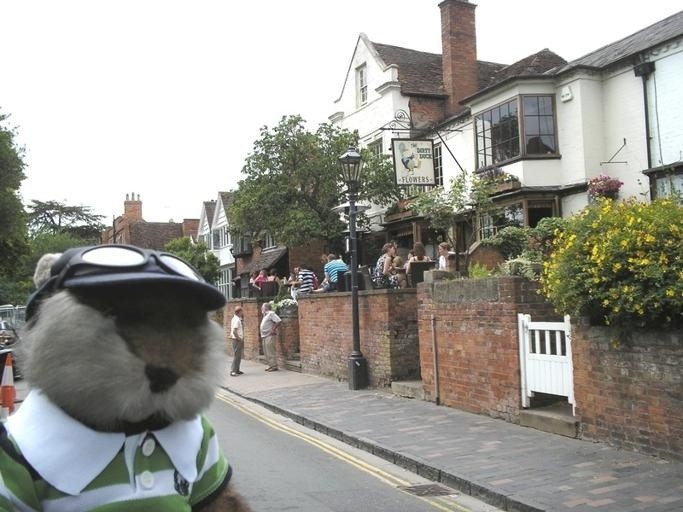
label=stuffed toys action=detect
[1,245,250,511]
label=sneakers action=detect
[230,370,244,376]
[265,366,278,372]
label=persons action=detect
[249,241,450,306]
[230,306,245,376]
[260,303,282,372]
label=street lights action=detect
[336,140,368,390]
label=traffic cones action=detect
[1,351,17,413]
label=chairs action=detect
[407,261,436,286]
[358,265,393,289]
[330,271,345,291]
[247,281,277,297]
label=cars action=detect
[0,306,29,379]
